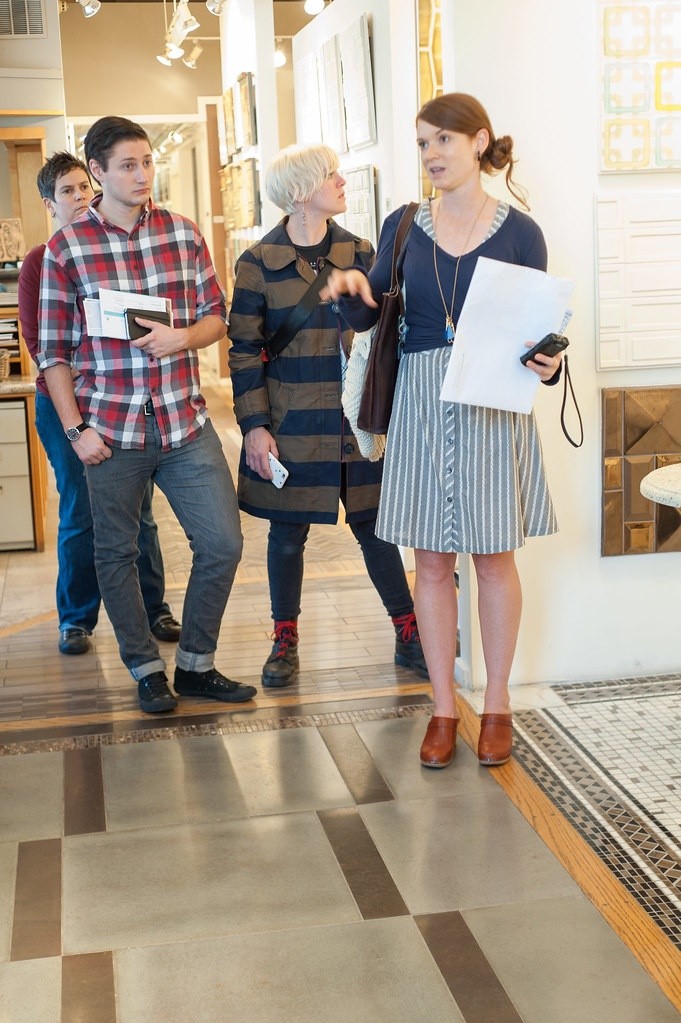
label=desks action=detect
[0,374,48,553]
[640,462,681,509]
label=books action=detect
[124,307,172,340]
[0,318,17,340]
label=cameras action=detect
[519,333,569,367]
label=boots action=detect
[388,605,430,680]
[261,616,300,687]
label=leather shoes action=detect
[419,715,461,767]
[478,713,513,766]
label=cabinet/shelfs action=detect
[0,401,35,553]
[0,306,30,380]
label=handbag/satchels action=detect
[356,201,420,436]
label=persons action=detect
[225,144,438,688]
[18,150,183,656]
[325,93,561,767]
[37,116,257,712]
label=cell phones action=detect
[268,451,289,489]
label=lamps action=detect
[68,0,342,69]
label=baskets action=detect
[0,349,10,382]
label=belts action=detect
[144,401,155,415]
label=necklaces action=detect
[433,192,489,344]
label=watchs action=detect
[67,421,90,442]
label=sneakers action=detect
[58,629,92,654]
[138,671,178,714]
[151,618,182,644]
[172,665,257,700]
